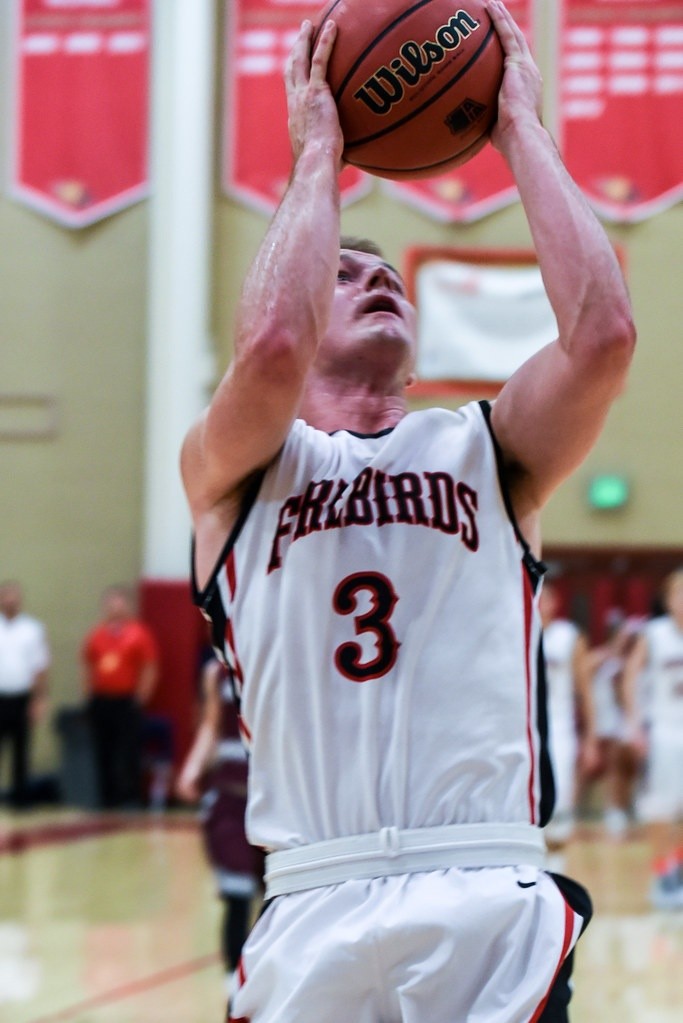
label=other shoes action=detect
[649,845,683,909]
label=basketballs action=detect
[312,0,506,182]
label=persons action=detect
[183,0,637,1023]
[0,581,51,807]
[177,660,268,973]
[538,569,683,907]
[77,586,158,808]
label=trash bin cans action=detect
[49,703,173,812]
